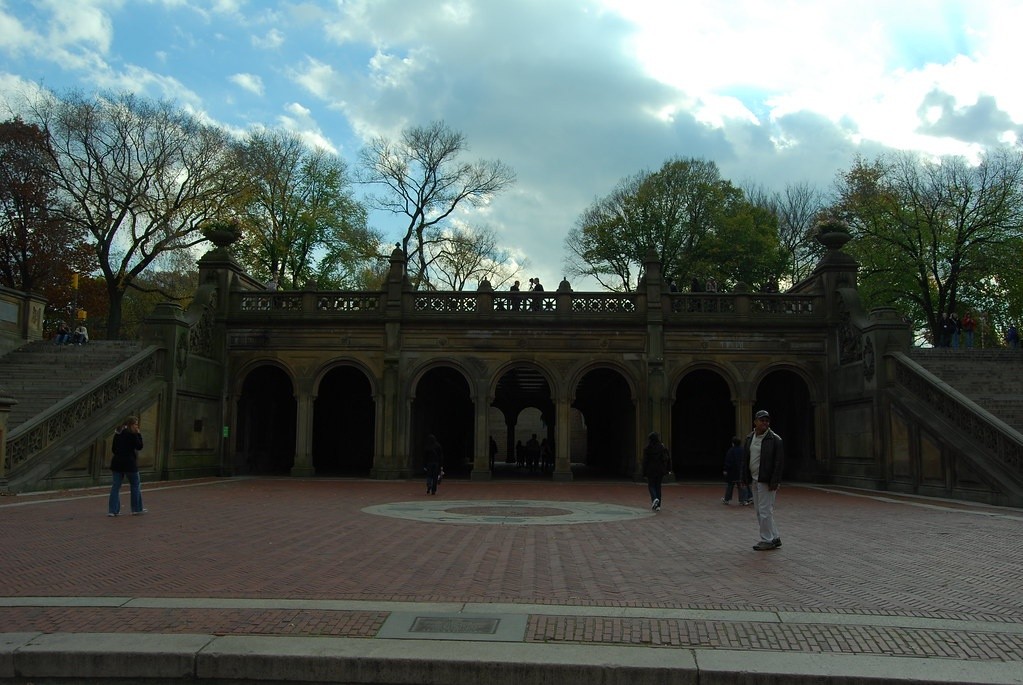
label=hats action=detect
[755,410,769,420]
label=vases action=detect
[821,232,851,249]
[206,230,241,246]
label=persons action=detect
[691,278,702,312]
[509,281,521,311]
[488,435,498,470]
[423,434,442,496]
[720,437,754,505]
[267,286,282,305]
[515,434,551,472]
[938,312,976,350]
[763,276,779,312]
[108,416,144,516]
[666,277,679,292]
[705,276,718,312]
[533,278,544,311]
[641,431,672,512]
[739,410,786,550]
[1004,325,1017,348]
[43,323,89,346]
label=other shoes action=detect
[132,508,148,516]
[427,486,431,494]
[655,506,660,511]
[651,498,659,510]
[738,499,748,506]
[109,510,120,516]
[753,537,782,550]
[721,497,729,505]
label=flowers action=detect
[812,217,857,243]
[198,216,242,232]
